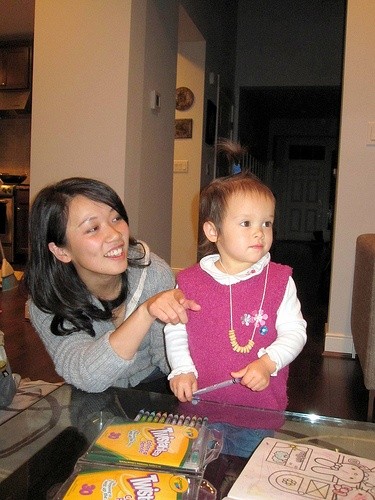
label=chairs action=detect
[350,233,375,421]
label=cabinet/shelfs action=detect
[0,40,33,91]
[15,190,28,264]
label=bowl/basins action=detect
[0,173,28,185]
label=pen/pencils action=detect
[139,408,197,427]
[133,407,145,422]
[194,414,203,431]
[191,416,208,462]
[191,376,241,396]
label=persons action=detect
[163,140,307,458]
[18,178,200,447]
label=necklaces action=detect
[218,251,270,354]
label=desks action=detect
[0,385,375,500]
